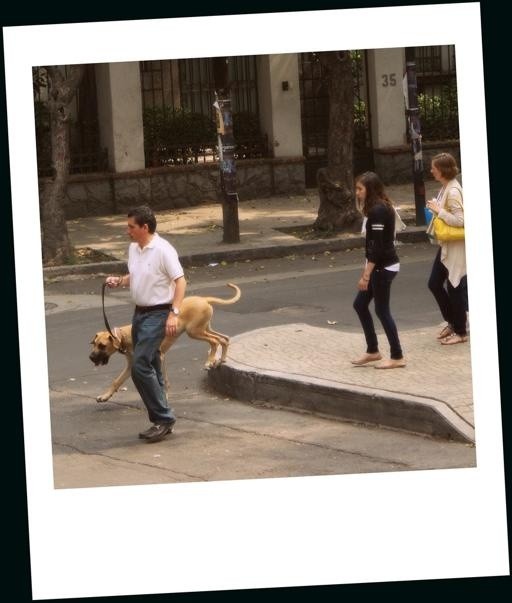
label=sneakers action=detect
[349,351,383,365]
[373,357,407,369]
[144,420,169,442]
[138,423,172,439]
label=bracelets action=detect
[118,274,123,286]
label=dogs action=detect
[88,282,241,403]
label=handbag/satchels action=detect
[431,216,466,242]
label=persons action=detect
[427,153,470,346]
[352,171,407,370]
[106,203,188,443]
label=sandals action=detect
[440,332,468,346]
[436,324,455,340]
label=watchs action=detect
[169,307,181,314]
